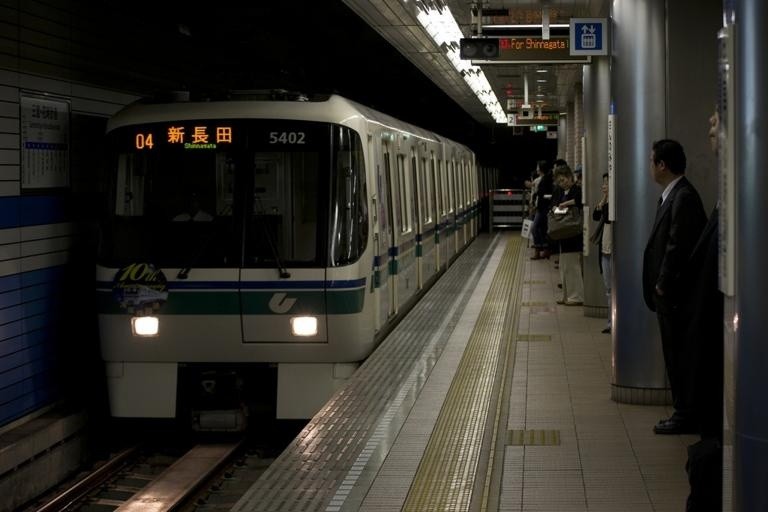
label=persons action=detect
[588,173,613,334]
[687,101,722,448]
[642,138,709,436]
[521,157,582,260]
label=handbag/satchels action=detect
[520,218,535,240]
[545,205,583,241]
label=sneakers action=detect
[652,411,698,437]
[529,249,583,307]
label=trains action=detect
[81,79,508,451]
